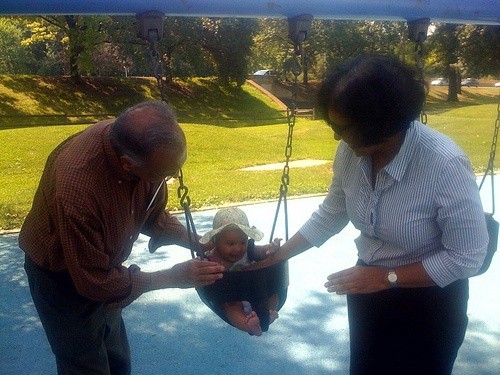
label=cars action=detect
[461,78,480,87]
[431,77,449,86]
[253,69,284,81]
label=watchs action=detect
[246,54,488,374]
[385,267,399,291]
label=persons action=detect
[19,101,223,374]
[196,206,289,337]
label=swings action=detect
[406,17,500,277]
[133,10,316,333]
